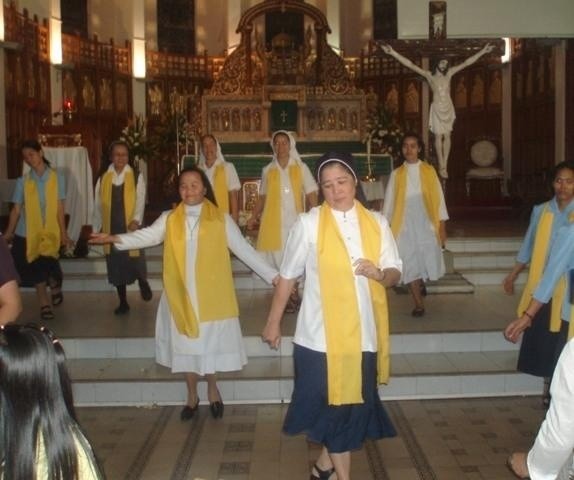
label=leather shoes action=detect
[420,279,427,296]
[412,307,425,317]
[114,303,129,314]
[139,281,152,301]
[181,397,200,420]
[210,389,224,418]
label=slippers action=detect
[507,451,530,479]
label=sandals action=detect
[40,305,54,320]
[310,463,335,480]
[50,283,62,305]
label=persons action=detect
[247,130,319,314]
[197,134,242,221]
[1,321,106,480]
[380,43,497,178]
[93,141,152,317]
[503,160,574,408]
[382,133,450,315]
[505,337,574,480]
[504,210,574,343]
[261,150,402,479]
[87,167,297,421]
[3,140,73,319]
[0,233,25,332]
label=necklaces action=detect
[184,217,201,244]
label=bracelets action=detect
[378,272,387,285]
[523,310,537,321]
